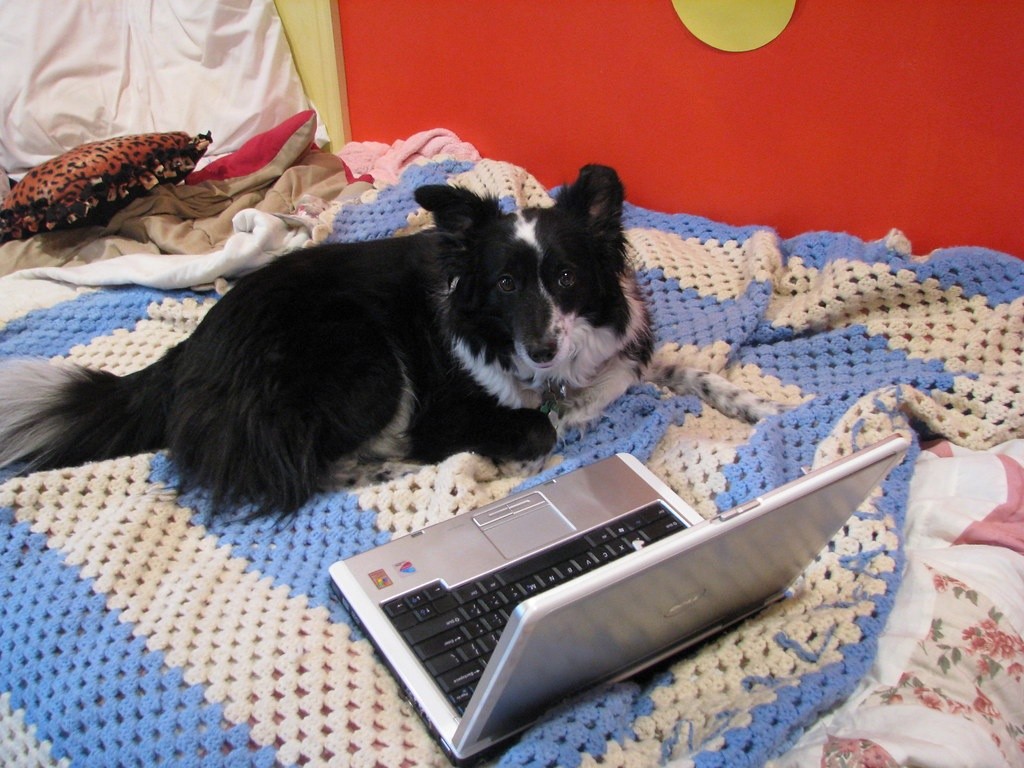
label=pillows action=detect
[0,129,214,244]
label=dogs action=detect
[0,163,659,540]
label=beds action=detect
[0,111,1024,768]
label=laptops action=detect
[327,430,909,768]
